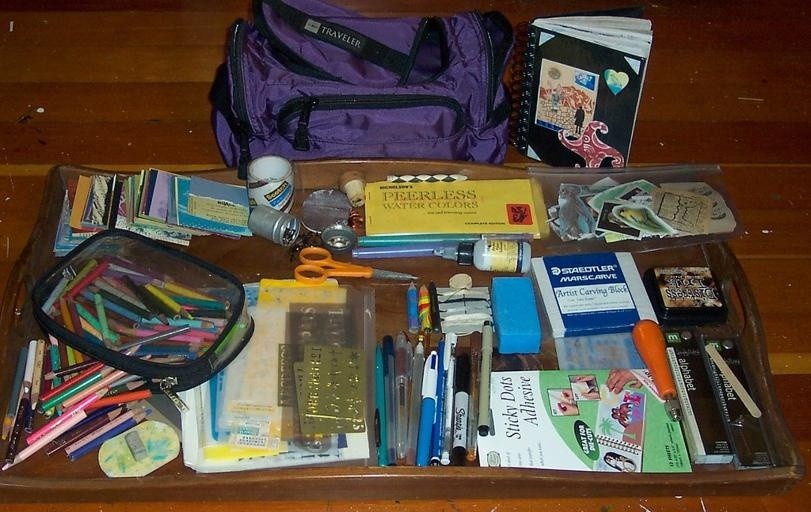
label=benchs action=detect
[2,0,811,389]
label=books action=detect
[515,15,655,168]
[54,168,253,258]
[477,368,693,475]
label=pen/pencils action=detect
[405,278,442,334]
[374,320,495,467]
[42,255,233,365]
[2,338,152,473]
[352,234,535,259]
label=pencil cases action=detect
[31,228,255,393]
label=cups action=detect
[246,153,297,216]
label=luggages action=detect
[205,1,517,170]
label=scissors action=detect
[294,246,420,284]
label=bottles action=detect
[432,237,533,275]
[248,203,301,247]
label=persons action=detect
[556,390,578,414]
[605,368,641,393]
[576,376,600,399]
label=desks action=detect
[1,156,804,504]
